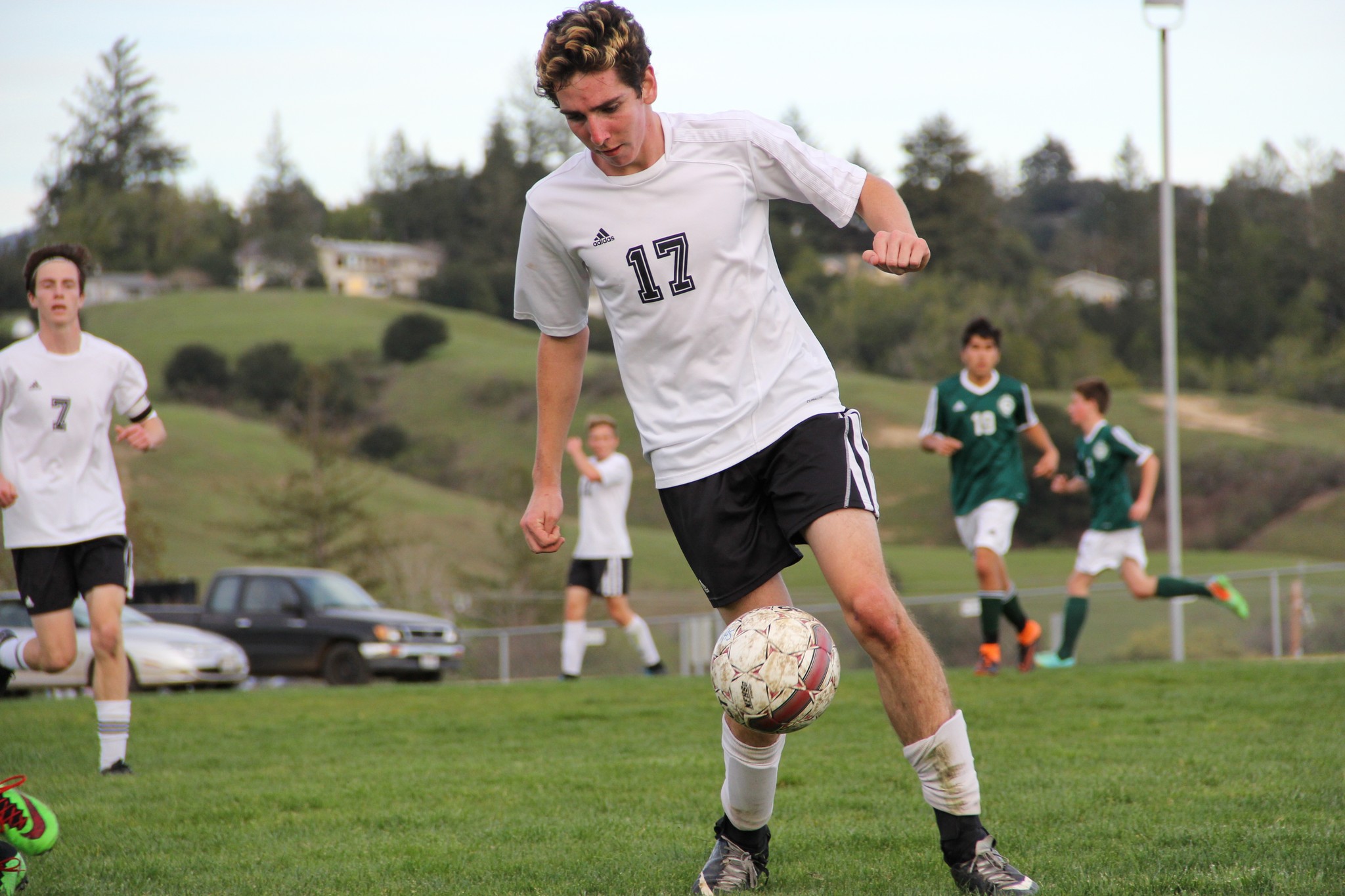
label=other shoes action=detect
[0,629,18,695]
[103,760,132,775]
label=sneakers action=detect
[1016,619,1041,672]
[691,818,771,895]
[0,840,29,896]
[0,776,60,856]
[1207,575,1250,622]
[1034,652,1074,669]
[973,641,1004,675]
[951,833,1039,894]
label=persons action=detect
[1,240,165,896]
[1029,379,1246,668]
[561,415,669,679]
[919,316,1060,682]
[513,1,1035,895]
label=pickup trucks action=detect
[127,565,465,688]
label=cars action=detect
[0,587,249,697]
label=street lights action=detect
[1138,2,1192,667]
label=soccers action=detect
[706,606,840,736]
